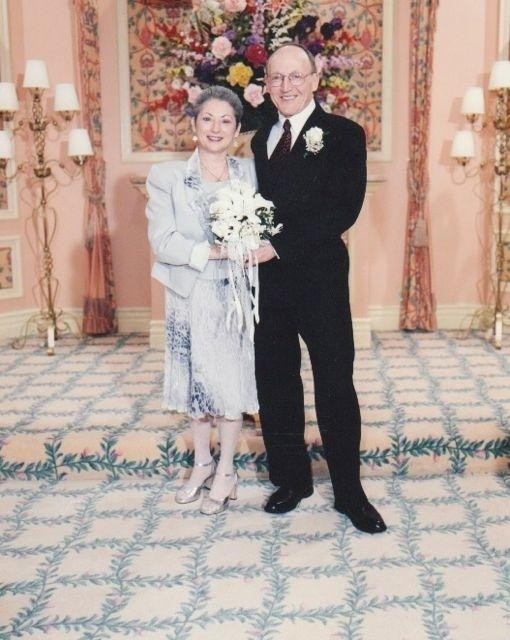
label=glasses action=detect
[270,72,312,86]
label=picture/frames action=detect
[115,0,396,162]
[2,234,25,298]
[0,155,17,218]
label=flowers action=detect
[205,180,284,264]
[148,1,364,123]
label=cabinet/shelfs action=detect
[131,176,390,350]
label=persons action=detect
[145,84,259,514]
[247,40,386,533]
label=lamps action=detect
[449,57,510,348]
[2,55,95,355]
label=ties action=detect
[269,120,290,162]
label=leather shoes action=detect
[265,486,313,513]
[334,499,386,532]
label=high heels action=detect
[201,467,237,514]
[175,457,216,503]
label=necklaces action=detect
[200,161,230,183]
